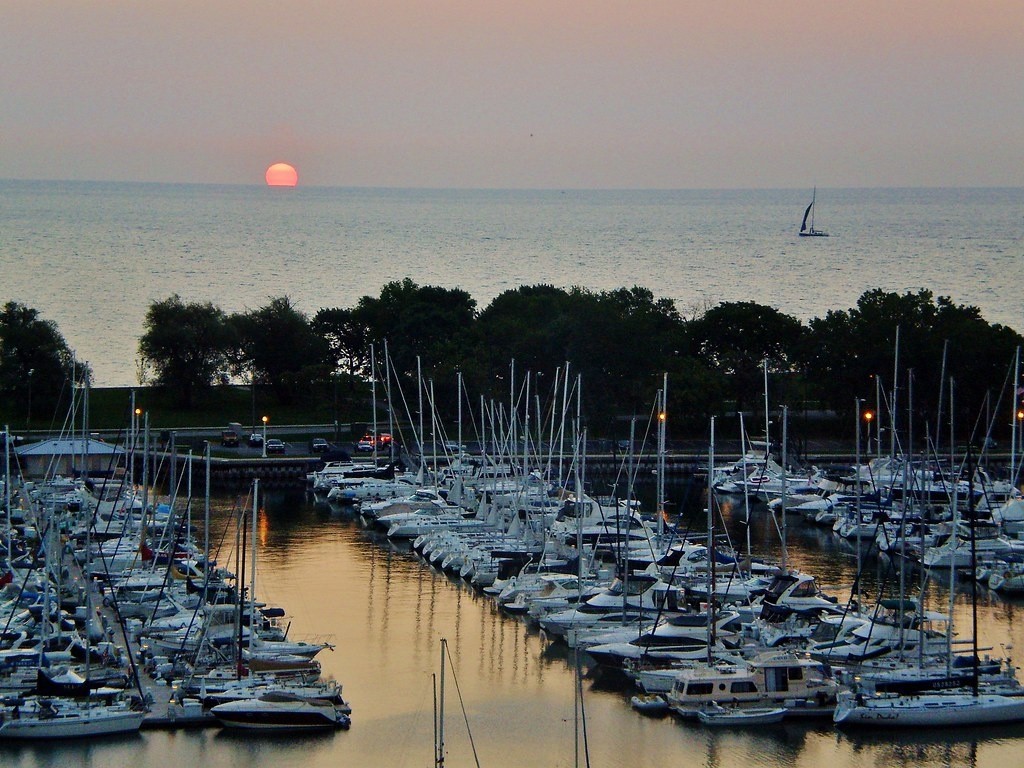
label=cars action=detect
[266,439,286,454]
[441,441,459,452]
[248,433,264,447]
[313,439,328,452]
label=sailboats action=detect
[0,349,338,742]
[298,340,1023,728]
[797,187,829,237]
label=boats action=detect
[211,691,337,734]
[631,693,668,714]
[710,323,1023,597]
[695,689,788,728]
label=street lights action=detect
[1018,413,1024,452]
[866,413,872,454]
[261,416,268,458]
[135,409,142,449]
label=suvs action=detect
[221,429,239,447]
